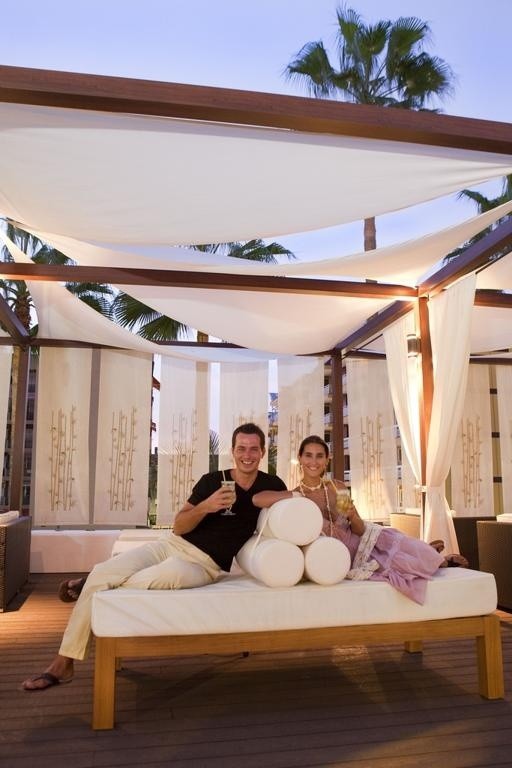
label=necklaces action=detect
[299,478,333,537]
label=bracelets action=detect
[293,489,300,500]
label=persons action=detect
[252,435,469,608]
[18,423,288,691]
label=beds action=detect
[91,527,505,732]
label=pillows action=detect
[304,535,352,586]
[0,510,20,524]
[496,512,512,522]
[257,497,324,546]
[236,538,305,587]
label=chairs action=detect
[389,510,497,568]
[476,519,512,609]
[0,516,30,613]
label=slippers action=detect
[442,551,469,568]
[429,539,446,552]
[22,667,73,692]
[58,576,85,603]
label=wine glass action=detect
[220,480,238,517]
[333,489,352,526]
